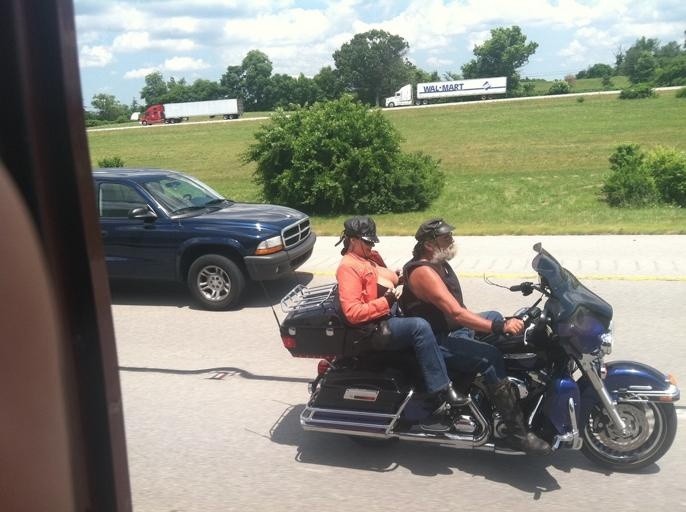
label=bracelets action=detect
[491,318,506,335]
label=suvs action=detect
[86,166,319,313]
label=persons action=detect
[332,215,474,410]
[396,216,552,459]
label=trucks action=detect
[140,97,243,126]
[384,76,508,109]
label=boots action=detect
[488,380,552,456]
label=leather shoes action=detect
[439,387,469,406]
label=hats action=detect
[343,217,380,244]
[415,219,455,240]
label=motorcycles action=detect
[273,237,682,476]
